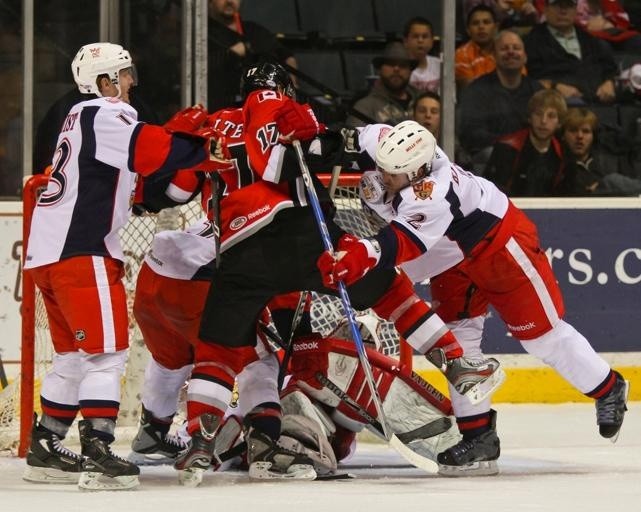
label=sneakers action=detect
[438,409,500,465]
[426,348,499,394]
[596,371,626,438]
[27,403,314,475]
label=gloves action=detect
[275,103,324,142]
[317,233,378,289]
[195,128,233,169]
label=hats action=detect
[373,32,418,70]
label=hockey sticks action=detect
[293,139,439,475]
[259,324,452,442]
[212,165,340,463]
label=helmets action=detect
[376,120,437,181]
[71,43,132,94]
[241,63,295,98]
